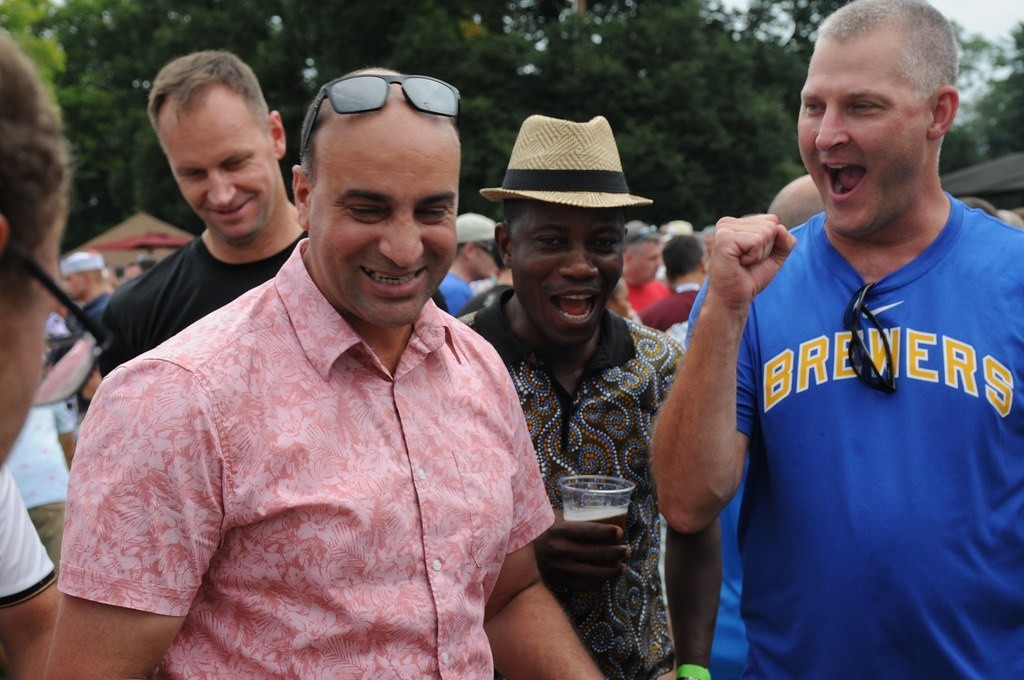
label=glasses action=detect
[10,247,111,407]
[302,73,461,149]
[843,283,898,394]
[630,225,659,242]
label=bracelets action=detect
[676,663,711,680]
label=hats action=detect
[59,251,103,275]
[478,114,654,209]
[661,220,694,243]
[455,212,496,243]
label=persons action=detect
[657,0,1024,680]
[44,202,1024,334]
[53,66,608,680]
[685,174,824,679]
[0,474,61,679]
[451,114,723,680]
[5,377,82,574]
[0,29,113,471]
[101,49,449,379]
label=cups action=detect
[557,475,636,542]
